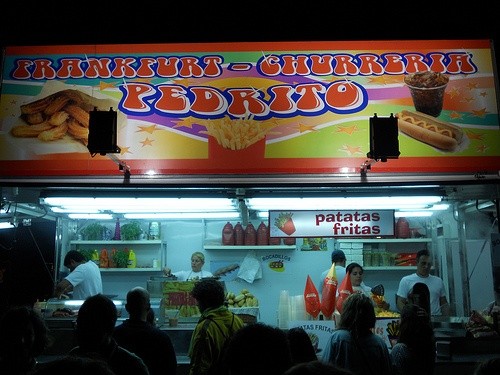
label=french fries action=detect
[11,91,91,141]
[194,113,281,151]
[273,211,294,228]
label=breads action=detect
[227,288,258,307]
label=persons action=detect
[0,307,112,375]
[321,294,393,373]
[396,248,450,317]
[336,263,386,314]
[202,323,354,375]
[318,249,347,315]
[390,307,438,370]
[113,290,177,375]
[189,280,248,375]
[56,250,103,299]
[65,295,149,375]
[163,252,214,281]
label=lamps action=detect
[0,218,18,229]
[87,108,131,176]
[360,111,402,173]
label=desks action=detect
[287,318,401,362]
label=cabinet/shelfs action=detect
[68,238,167,274]
[333,236,435,271]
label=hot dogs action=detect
[395,110,464,149]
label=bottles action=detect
[243,222,257,245]
[396,218,409,238]
[127,249,136,268]
[269,226,281,245]
[255,221,268,245]
[108,249,117,268]
[99,248,109,268]
[90,249,101,267]
[284,237,296,245]
[222,221,235,245]
[233,222,244,245]
[124,248,128,253]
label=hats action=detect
[332,250,347,261]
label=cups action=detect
[153,259,160,268]
[409,229,417,238]
[278,289,310,330]
[362,252,392,266]
[404,76,449,117]
